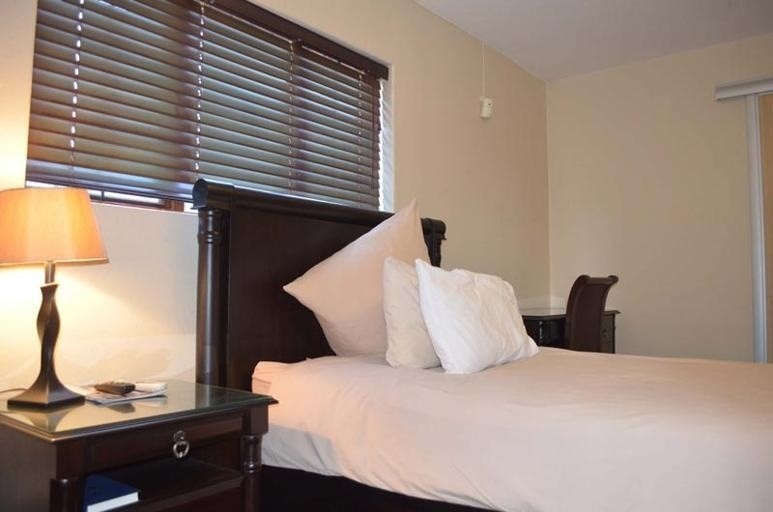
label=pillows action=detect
[284,197,431,363]
[416,257,541,374]
[381,256,443,369]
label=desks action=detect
[521,309,622,353]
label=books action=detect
[81,475,141,511]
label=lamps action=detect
[0,185,110,411]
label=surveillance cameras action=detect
[478,94,495,123]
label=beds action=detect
[193,176,773,511]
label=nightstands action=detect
[2,381,277,511]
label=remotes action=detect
[93,381,135,395]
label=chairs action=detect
[556,275,619,354]
